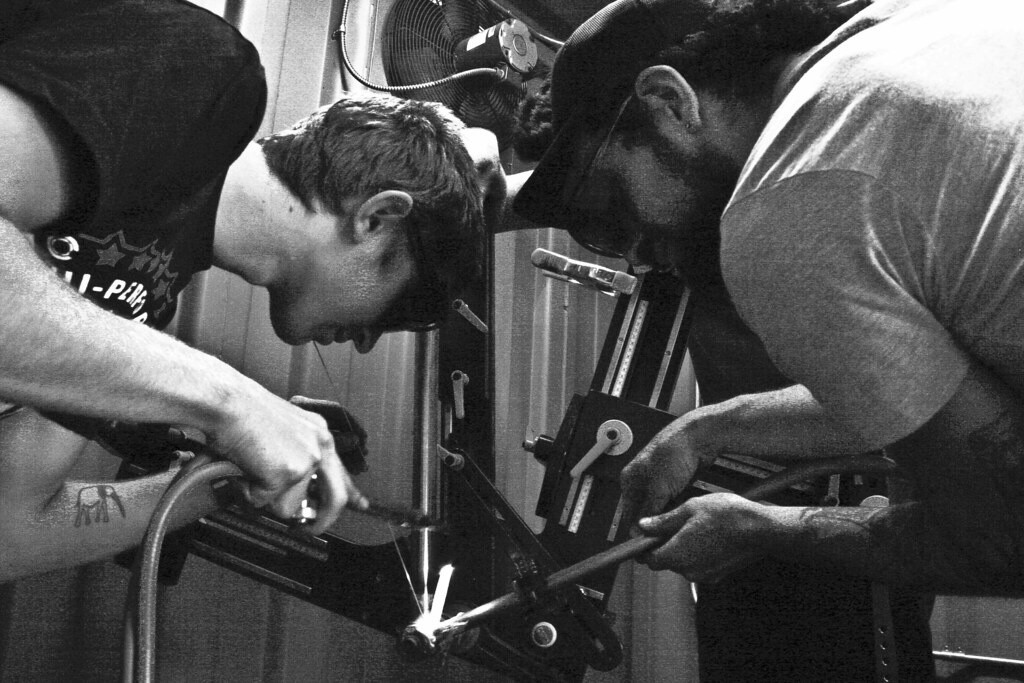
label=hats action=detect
[514,0,712,227]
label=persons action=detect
[0,0,486,583]
[513,2,1024,590]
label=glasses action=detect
[400,210,448,332]
[565,88,638,259]
